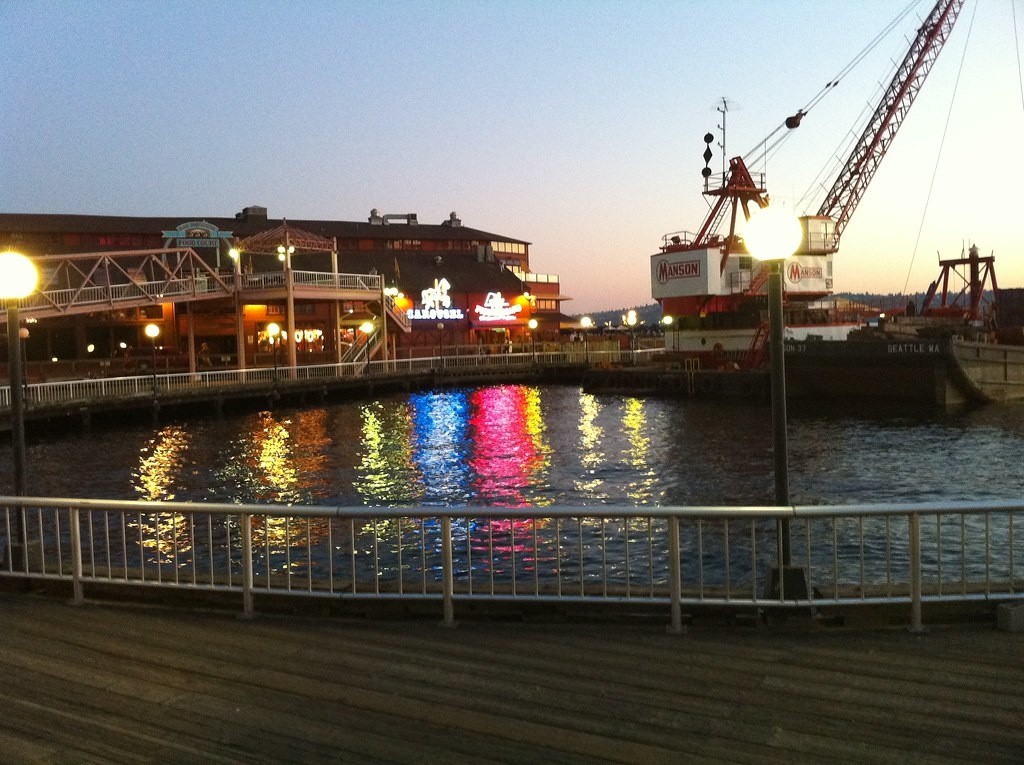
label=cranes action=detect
[649,1,965,325]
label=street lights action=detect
[581,316,591,367]
[267,322,281,390]
[0,248,40,567]
[626,315,638,367]
[527,318,538,369]
[141,322,162,408]
[362,321,374,380]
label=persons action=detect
[574,331,580,342]
[124,345,137,371]
[905,301,915,318]
[501,337,509,365]
[198,342,213,368]
[478,343,492,365]
